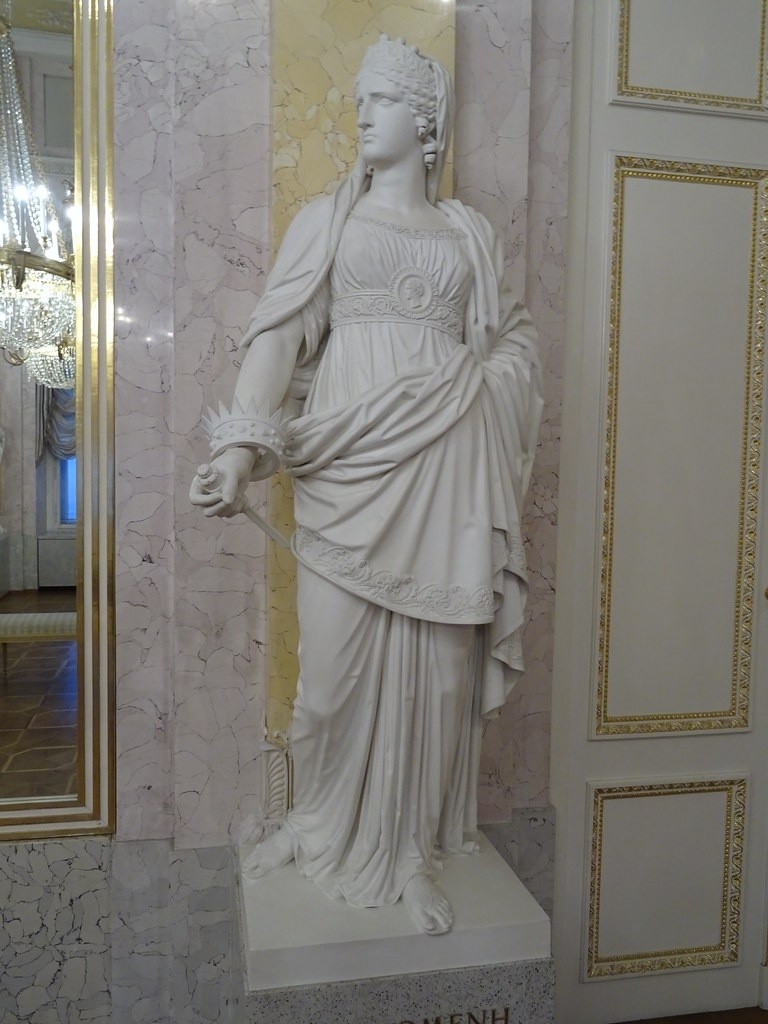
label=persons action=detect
[188,34,544,938]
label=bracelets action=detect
[200,395,298,482]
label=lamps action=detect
[0,12,76,389]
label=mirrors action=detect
[0,0,119,842]
